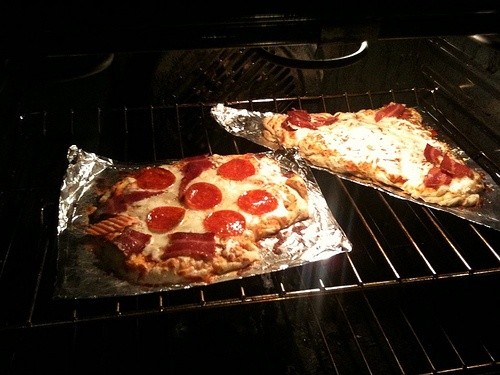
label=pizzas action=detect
[87,152,309,285]
[260,101,485,207]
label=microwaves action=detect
[0,1,500,375]
